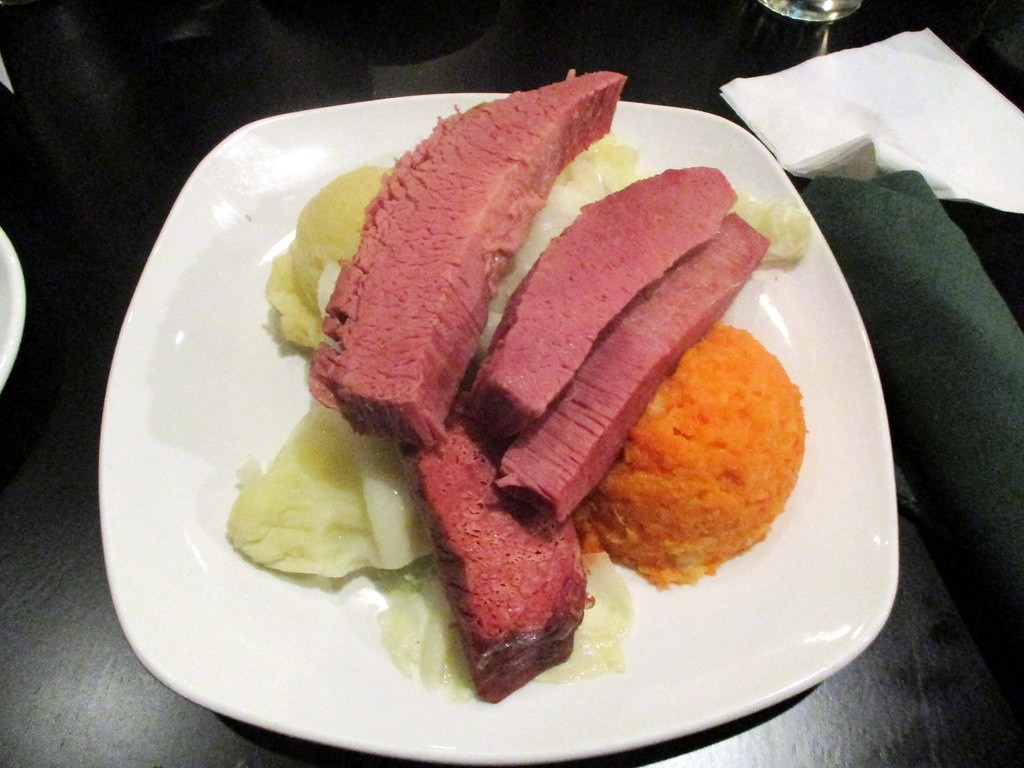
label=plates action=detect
[99,93,898,767]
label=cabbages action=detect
[221,131,810,700]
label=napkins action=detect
[719,28,1024,215]
